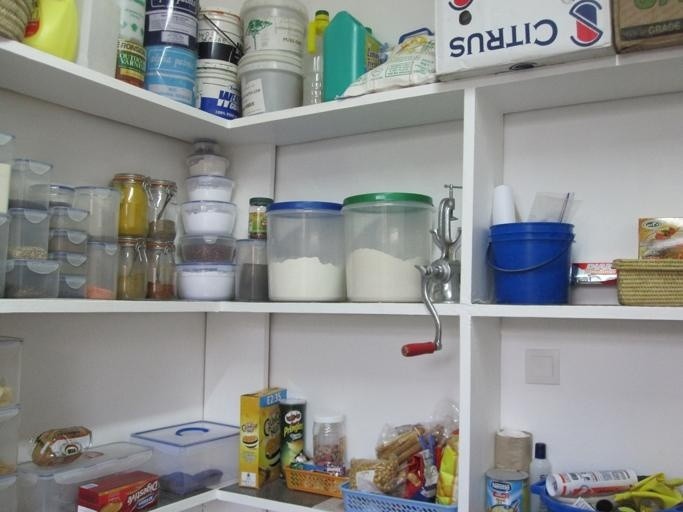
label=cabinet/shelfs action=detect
[1,38,683,511]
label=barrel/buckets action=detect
[195,10,242,65]
[237,50,304,117]
[241,0,307,55]
[193,58,241,120]
